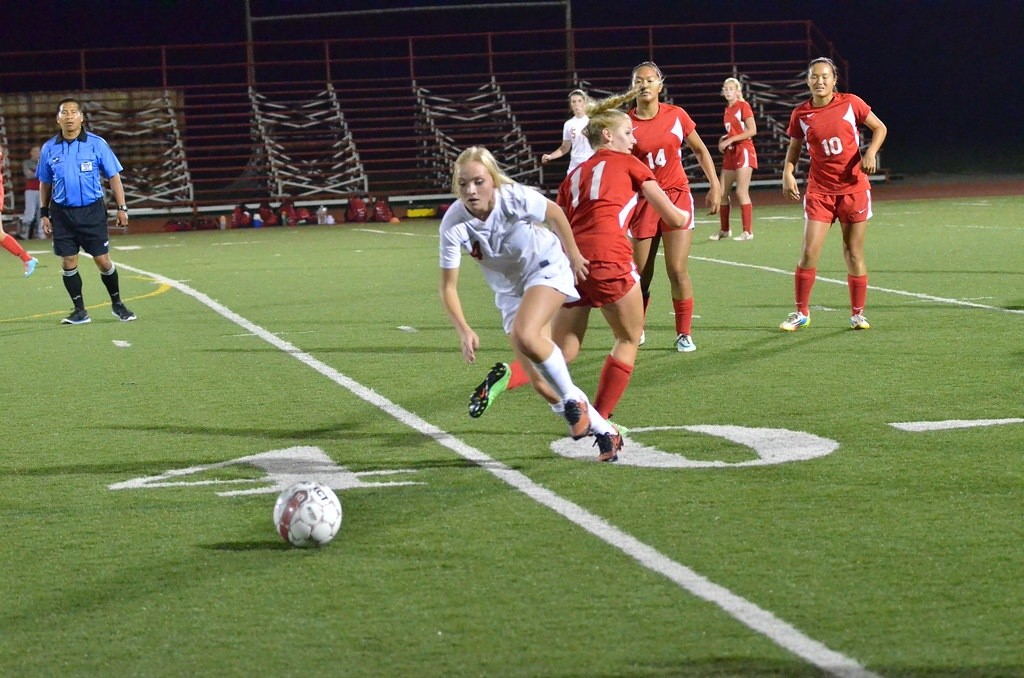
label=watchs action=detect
[117,205,129,212]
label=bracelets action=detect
[39,206,49,218]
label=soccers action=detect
[273,480,344,549]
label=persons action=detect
[20,145,47,240]
[438,145,629,462]
[626,61,722,353]
[35,97,138,325]
[538,87,595,176]
[467,82,689,419]
[710,77,758,242]
[777,56,889,332]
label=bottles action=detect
[281,211,287,225]
[220,216,226,229]
[438,205,448,216]
[252,214,263,227]
[317,204,327,224]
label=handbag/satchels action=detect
[165,219,192,231]
[296,208,317,223]
[196,219,221,230]
[260,206,278,226]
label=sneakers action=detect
[61,309,92,324]
[592,425,624,463]
[734,231,754,240]
[640,331,645,346]
[24,257,39,278]
[709,229,732,240]
[112,304,137,321]
[468,363,511,418]
[564,400,590,440]
[605,414,628,435]
[779,311,810,330]
[851,315,872,329]
[673,333,696,352]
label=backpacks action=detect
[345,197,368,222]
[277,200,296,225]
[373,201,392,222]
